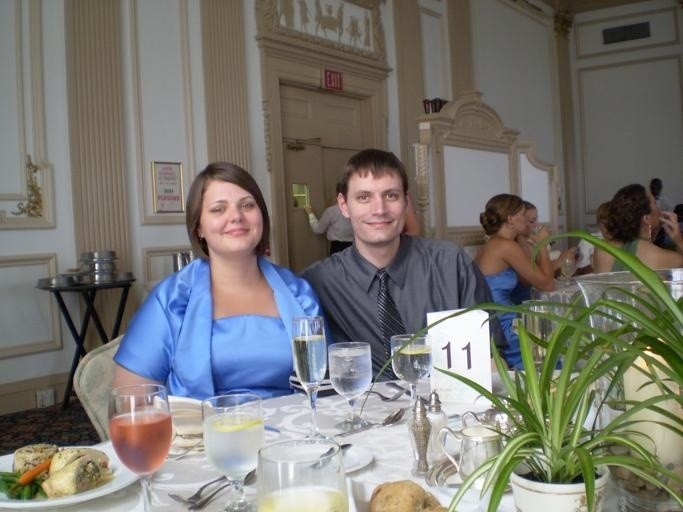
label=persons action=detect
[299,149,511,382]
[576,202,610,275]
[649,178,683,251]
[595,182,683,336]
[480,194,562,369]
[516,201,578,273]
[110,161,333,413]
[304,180,354,257]
[401,202,420,236]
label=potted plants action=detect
[354,280,681,512]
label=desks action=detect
[35,277,132,412]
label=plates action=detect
[0,444,140,511]
[346,441,371,474]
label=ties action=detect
[376,270,407,380]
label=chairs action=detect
[63,331,124,442]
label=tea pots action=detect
[460,399,527,455]
[575,271,683,489]
[437,409,509,488]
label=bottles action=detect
[428,389,446,461]
[409,394,434,475]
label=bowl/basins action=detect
[33,250,136,290]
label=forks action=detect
[360,389,406,402]
[168,463,257,511]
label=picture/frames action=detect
[149,159,184,215]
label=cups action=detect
[256,438,350,511]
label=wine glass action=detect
[389,333,430,415]
[198,392,262,510]
[291,317,331,450]
[108,383,172,512]
[324,341,374,432]
[518,299,551,379]
[557,252,577,287]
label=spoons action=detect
[335,405,407,438]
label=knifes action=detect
[308,443,352,469]
[386,380,428,404]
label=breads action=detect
[369,479,451,512]
[12,442,110,497]
